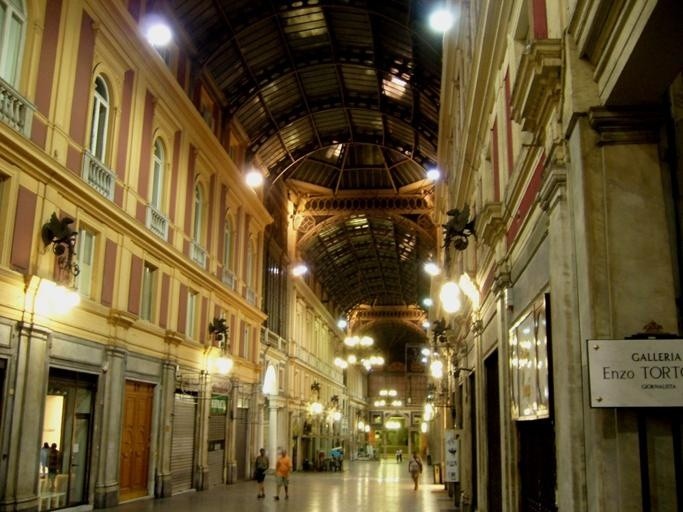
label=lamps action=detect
[429,316,451,379]
[310,380,324,415]
[26,210,82,321]
[203,319,234,376]
[331,394,343,422]
[438,202,480,314]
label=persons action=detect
[271,447,292,500]
[395,448,402,463]
[254,448,268,498]
[44,442,58,491]
[38,441,50,479]
[407,450,423,491]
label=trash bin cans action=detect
[427,455,432,466]
